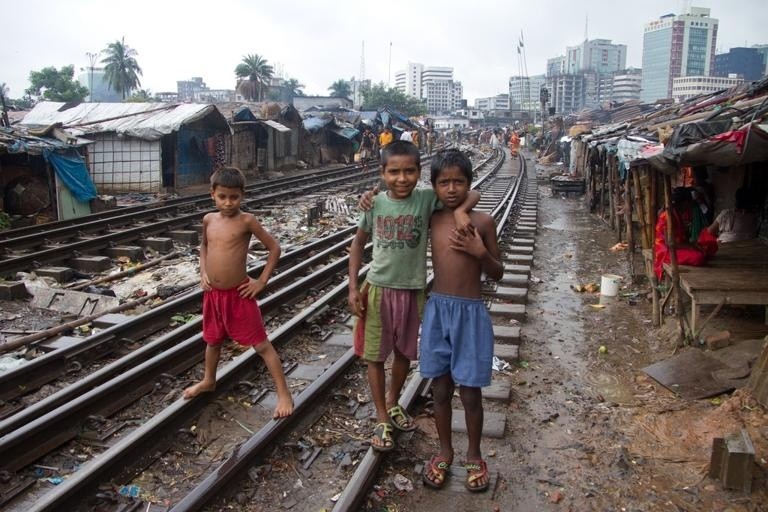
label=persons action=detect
[181,166,295,420]
[655,186,757,266]
[349,139,481,452]
[356,123,522,174]
[615,183,637,216]
[357,148,504,492]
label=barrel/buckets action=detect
[599,274,625,298]
[354,154,362,162]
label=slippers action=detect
[464,454,489,492]
[421,449,454,488]
[371,420,395,452]
[385,405,419,433]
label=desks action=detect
[661,260,767,344]
